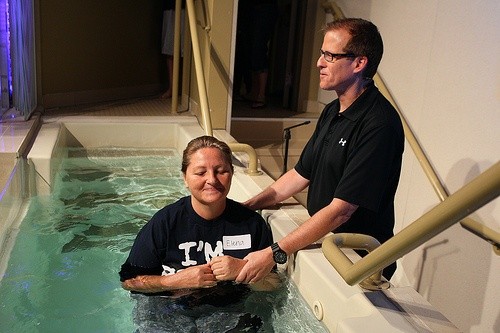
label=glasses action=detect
[319,49,355,62]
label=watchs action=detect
[271,242,287,265]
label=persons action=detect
[235,17,398,290]
[117,136,290,295]
[156,0,186,100]
[239,0,280,109]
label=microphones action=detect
[283,121,311,130]
